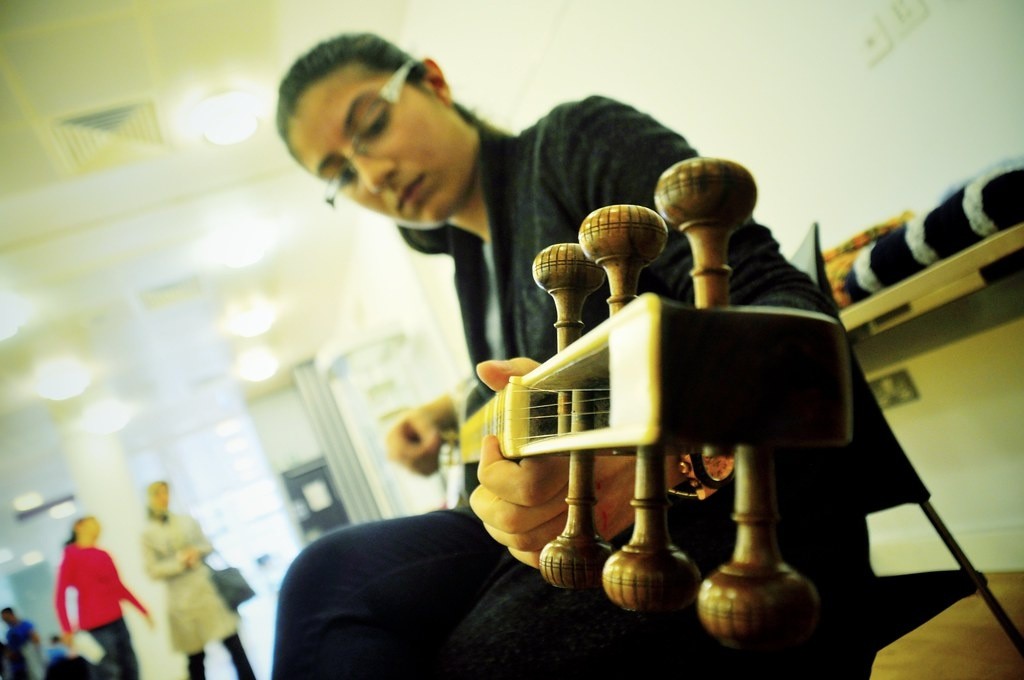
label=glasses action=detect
[324,60,414,207]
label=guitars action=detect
[434,157,855,652]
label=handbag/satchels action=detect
[204,548,255,610]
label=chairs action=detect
[787,221,1024,680]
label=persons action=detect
[0,607,67,680]
[135,478,257,679]
[55,515,153,680]
[273,32,883,679]
